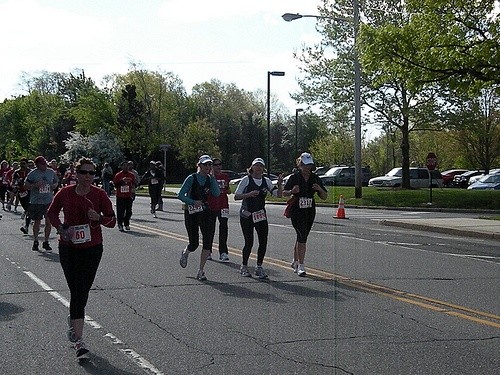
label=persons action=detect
[93,163,114,196]
[283,153,327,276]
[47,158,116,356]
[234,158,284,280]
[0,157,37,234]
[24,156,59,250]
[139,161,166,217]
[47,159,78,195]
[177,155,220,281]
[114,161,136,232]
[208,159,232,261]
[128,161,138,220]
[156,161,164,211]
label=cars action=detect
[220,170,292,187]
[453,170,486,188]
[440,169,474,188]
[467,170,500,191]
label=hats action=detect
[299,152,315,165]
[199,155,213,164]
[252,158,265,167]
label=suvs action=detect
[318,166,371,187]
[368,167,445,188]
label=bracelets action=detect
[290,190,292,194]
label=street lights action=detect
[267,70,287,175]
[295,108,304,168]
[281,10,365,199]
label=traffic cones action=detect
[334,195,349,219]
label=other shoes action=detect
[155,207,163,211]
[297,263,307,276]
[118,224,124,232]
[239,264,251,277]
[42,241,53,250]
[180,251,188,268]
[66,314,78,343]
[197,270,208,281]
[220,252,230,261]
[253,266,269,279]
[291,259,298,273]
[207,254,213,260]
[32,240,39,250]
[20,227,28,234]
[73,338,90,358]
[151,211,158,218]
[125,224,130,230]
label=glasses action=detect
[78,169,96,176]
[203,163,212,166]
[214,163,222,166]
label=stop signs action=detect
[426,152,437,170]
